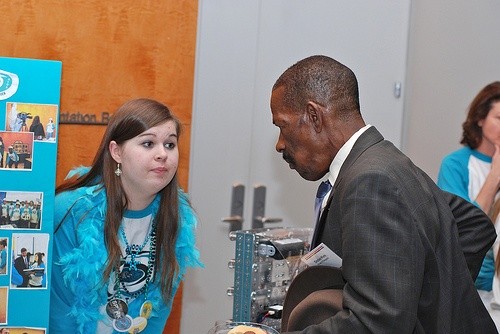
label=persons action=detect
[4,145,20,169]
[49,97,205,334]
[268,54,500,334]
[31,115,55,141]
[0,197,41,229]
[436,80,500,334]
[0,240,8,275]
[12,248,46,288]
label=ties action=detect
[309,179,332,251]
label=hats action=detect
[281,266,346,332]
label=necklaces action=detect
[114,217,156,305]
